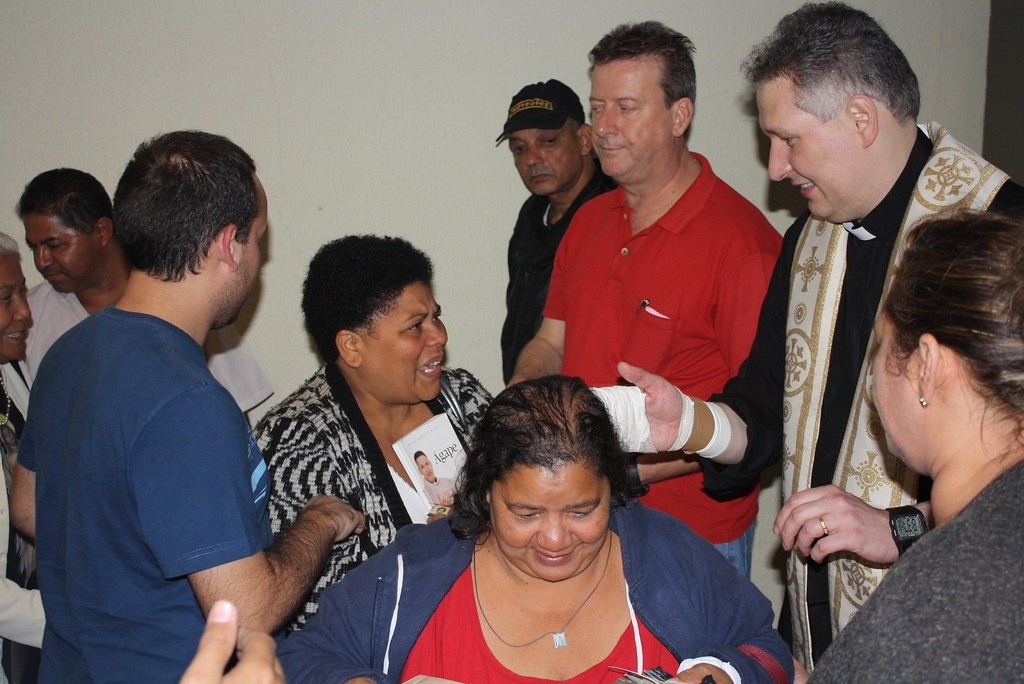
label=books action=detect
[392,413,470,513]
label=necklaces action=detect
[0,377,11,425]
[474,529,611,648]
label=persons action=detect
[255,235,496,632]
[803,217,1024,684]
[511,21,786,581]
[414,450,456,506]
[276,375,796,684]
[588,1,1024,675]
[0,167,289,683]
[12,130,366,683]
[496,80,615,389]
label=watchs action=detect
[885,505,928,557]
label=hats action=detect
[495,79,580,141]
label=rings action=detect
[819,517,828,535]
